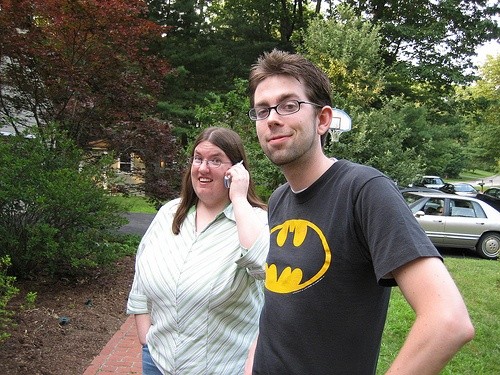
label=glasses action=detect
[249,101,324,120]
[191,156,234,167]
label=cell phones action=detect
[224,159,244,189]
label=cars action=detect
[400,192,500,258]
[440,183,480,196]
[400,189,445,195]
[476,187,500,213]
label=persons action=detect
[125,126,272,375]
[247,47,475,375]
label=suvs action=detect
[413,174,445,189]
